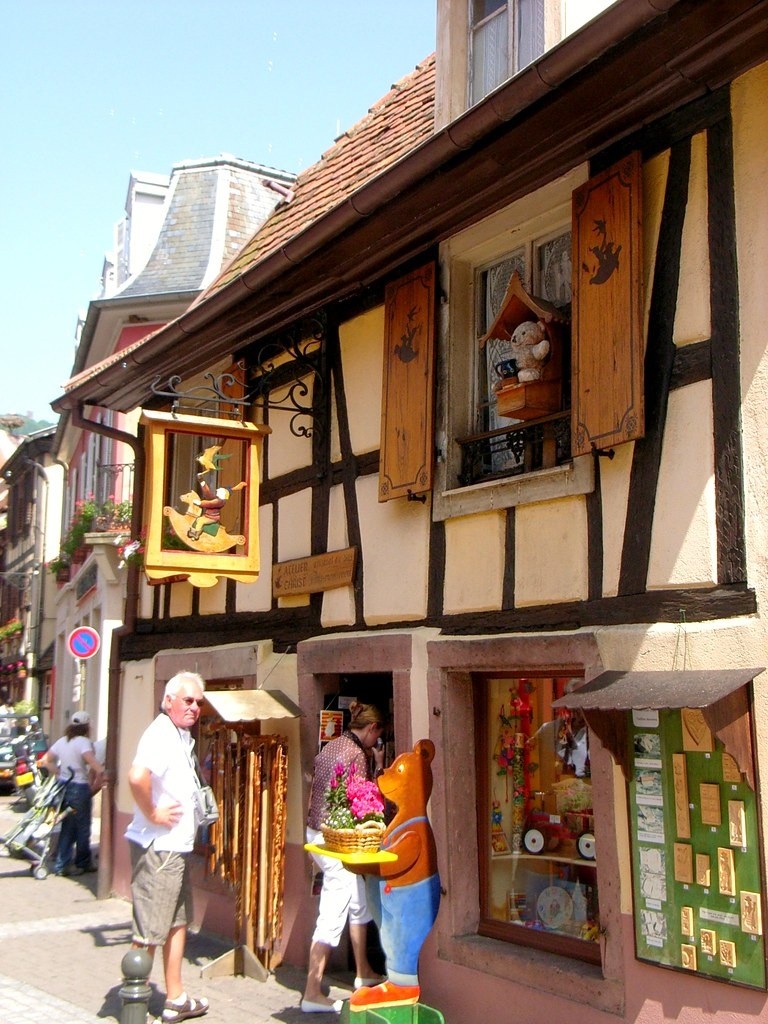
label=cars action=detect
[0,714,48,790]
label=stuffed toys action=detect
[493,322,550,390]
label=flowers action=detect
[60,491,133,547]
[0,665,5,673]
[16,660,24,668]
[321,753,385,828]
[7,663,16,672]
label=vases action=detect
[18,669,27,680]
[0,675,7,682]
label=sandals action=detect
[162,995,210,1023]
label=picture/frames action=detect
[492,832,511,856]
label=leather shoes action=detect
[353,975,387,990]
[301,999,344,1013]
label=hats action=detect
[70,711,91,725]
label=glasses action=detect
[180,697,205,707]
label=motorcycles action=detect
[4,714,43,808]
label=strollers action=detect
[0,765,77,880]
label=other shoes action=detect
[78,865,98,872]
[57,868,83,877]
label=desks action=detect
[491,851,596,887]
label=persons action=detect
[42,711,103,876]
[301,700,386,1013]
[29,716,50,760]
[123,670,209,1024]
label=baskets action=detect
[320,820,385,854]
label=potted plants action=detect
[71,517,131,563]
[7,673,17,681]
[49,557,71,583]
[0,621,22,643]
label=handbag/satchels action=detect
[194,784,218,828]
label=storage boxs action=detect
[565,810,588,834]
[544,793,556,814]
